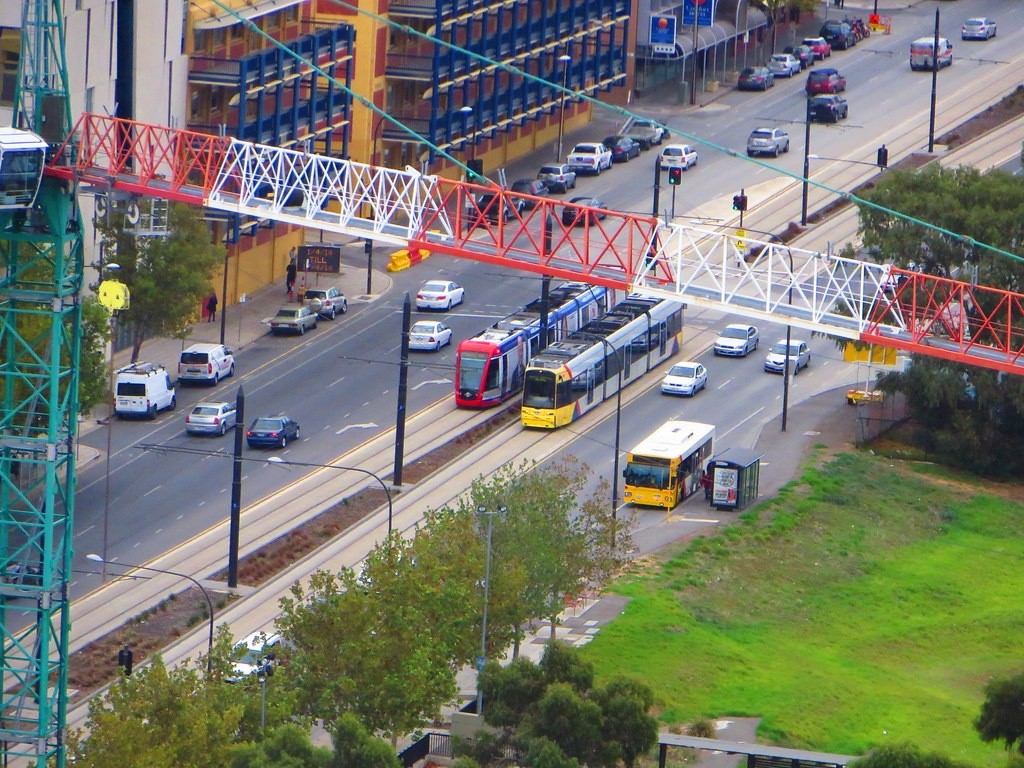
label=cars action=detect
[880,263,922,292]
[313,548,419,607]
[763,339,812,375]
[714,324,760,358]
[737,14,1000,156]
[112,119,699,449]
[661,361,708,398]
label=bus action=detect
[521,292,688,429]
[623,419,716,508]
[454,272,631,409]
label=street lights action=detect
[87,552,214,692]
[497,321,621,548]
[476,503,507,714]
[689,219,793,431]
[268,456,393,563]
[557,19,604,164]
[367,105,472,294]
[467,55,571,232]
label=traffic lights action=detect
[733,195,744,210]
[669,167,681,184]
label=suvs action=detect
[223,630,282,686]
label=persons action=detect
[285,259,296,294]
[290,247,297,265]
[206,293,218,323]
